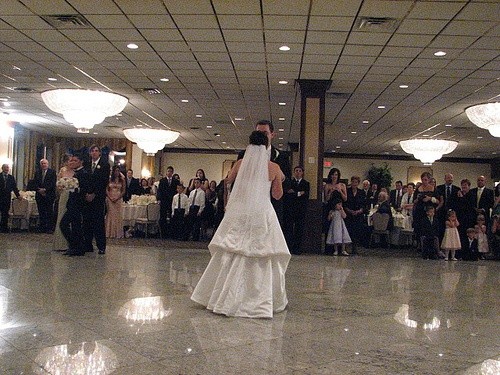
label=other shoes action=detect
[444,258,448,261]
[451,257,457,261]
[62,245,105,256]
[342,250,349,256]
[333,251,338,255]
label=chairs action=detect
[399,228,417,248]
[369,213,391,247]
[11,197,29,233]
[135,203,160,239]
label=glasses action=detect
[296,170,301,172]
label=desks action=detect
[122,203,159,238]
[8,190,39,232]
[367,211,412,246]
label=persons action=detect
[190,120,291,318]
[0,164,22,232]
[105,164,217,242]
[324,168,500,260]
[34,159,57,233]
[21,243,500,354]
[287,166,310,254]
[53,144,109,256]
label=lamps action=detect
[123,128,180,156]
[399,139,458,165]
[41,88,129,133]
[465,102,500,137]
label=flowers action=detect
[56,176,79,191]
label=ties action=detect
[191,190,197,208]
[397,190,400,205]
[92,163,96,174]
[297,180,299,186]
[4,175,8,188]
[178,194,181,214]
[446,187,450,197]
